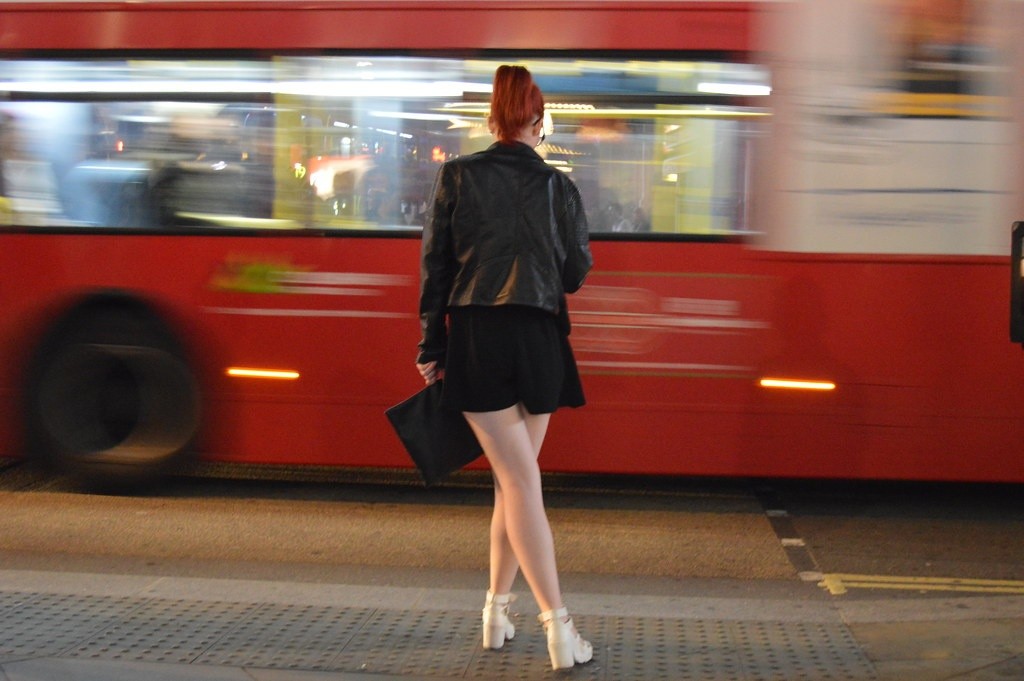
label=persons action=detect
[414,66,593,670]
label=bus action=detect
[0,2,1024,495]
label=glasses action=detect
[533,117,546,147]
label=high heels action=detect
[482,589,516,649]
[537,608,595,670]
